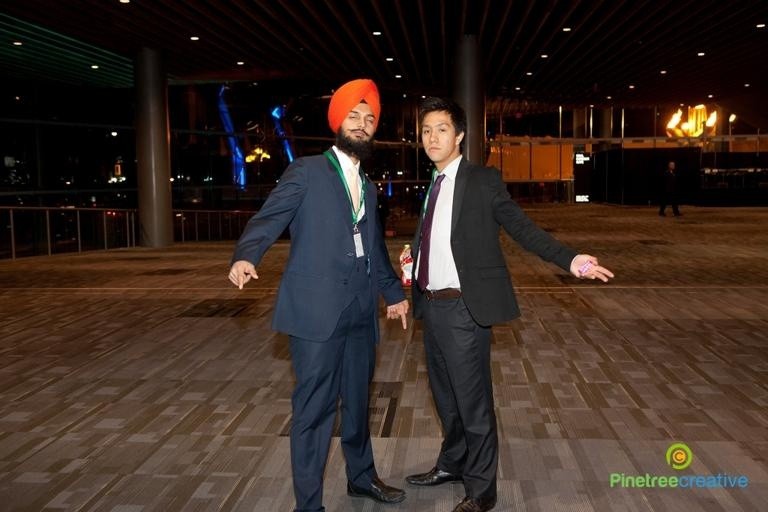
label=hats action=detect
[327,78,381,134]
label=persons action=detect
[404,95,615,512]
[658,160,684,217]
[227,79,408,512]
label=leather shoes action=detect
[453,497,497,512]
[406,467,463,486]
[347,479,406,503]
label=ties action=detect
[417,175,445,292]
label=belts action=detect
[426,289,460,300]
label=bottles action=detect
[400,242,412,289]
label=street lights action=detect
[244,145,271,195]
